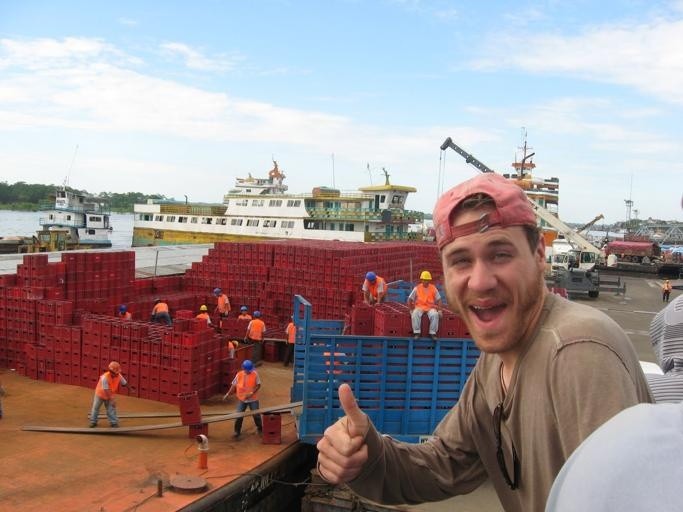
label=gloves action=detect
[244,337,249,344]
[409,310,414,317]
[438,310,442,319]
[245,392,253,402]
[222,393,230,403]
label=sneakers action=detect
[414,334,420,341]
[431,333,438,342]
[89,422,96,429]
[112,422,118,429]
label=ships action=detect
[129,161,435,248]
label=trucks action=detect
[604,242,659,263]
[291,281,664,444]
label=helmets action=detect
[120,305,127,314]
[242,360,254,372]
[199,305,207,311]
[152,298,161,305]
[214,288,222,295]
[240,306,248,311]
[365,271,377,282]
[420,271,433,282]
[253,311,262,318]
[107,362,124,375]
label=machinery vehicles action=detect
[437,137,626,298]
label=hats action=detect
[432,174,538,251]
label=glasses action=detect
[490,401,519,492]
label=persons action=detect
[544,403,683,510]
[407,271,442,341]
[89,361,133,428]
[315,173,656,511]
[222,361,263,438]
[227,341,238,360]
[662,280,672,301]
[212,288,231,331]
[361,272,386,307]
[197,306,211,327]
[283,318,297,365]
[239,307,251,321]
[244,311,266,365]
[117,306,131,322]
[149,299,171,325]
[644,295,683,405]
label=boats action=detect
[660,227,683,248]
[0,179,112,250]
